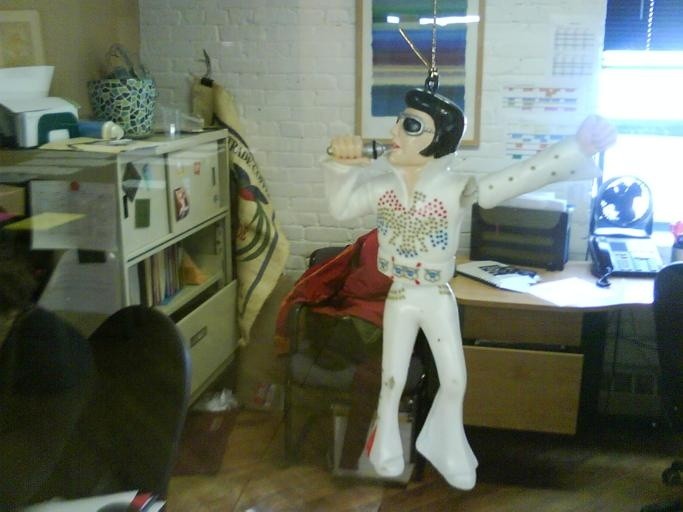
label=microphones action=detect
[325,139,385,159]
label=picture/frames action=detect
[354,0,484,147]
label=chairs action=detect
[639,263,682,511]
[283,246,428,483]
[87,305,192,511]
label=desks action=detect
[448,256,683,436]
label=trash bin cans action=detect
[174,386,244,477]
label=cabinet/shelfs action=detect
[0,127,241,415]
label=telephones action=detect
[587,234,665,287]
